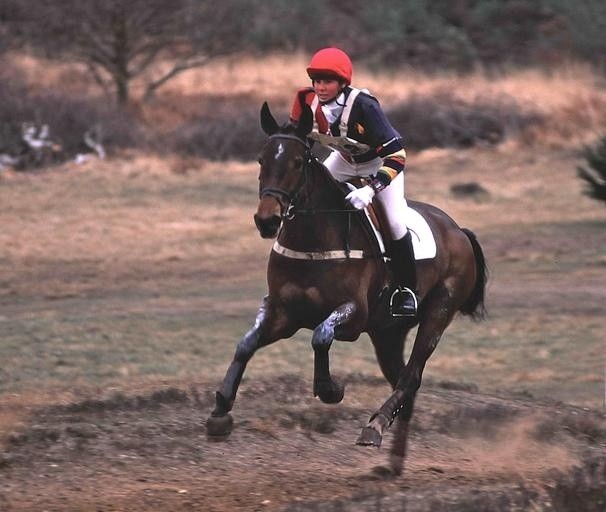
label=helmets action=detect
[307,46,353,85]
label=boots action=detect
[388,231,419,316]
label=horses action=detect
[205,100,491,481]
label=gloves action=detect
[345,185,375,209]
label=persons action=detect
[289,47,419,316]
